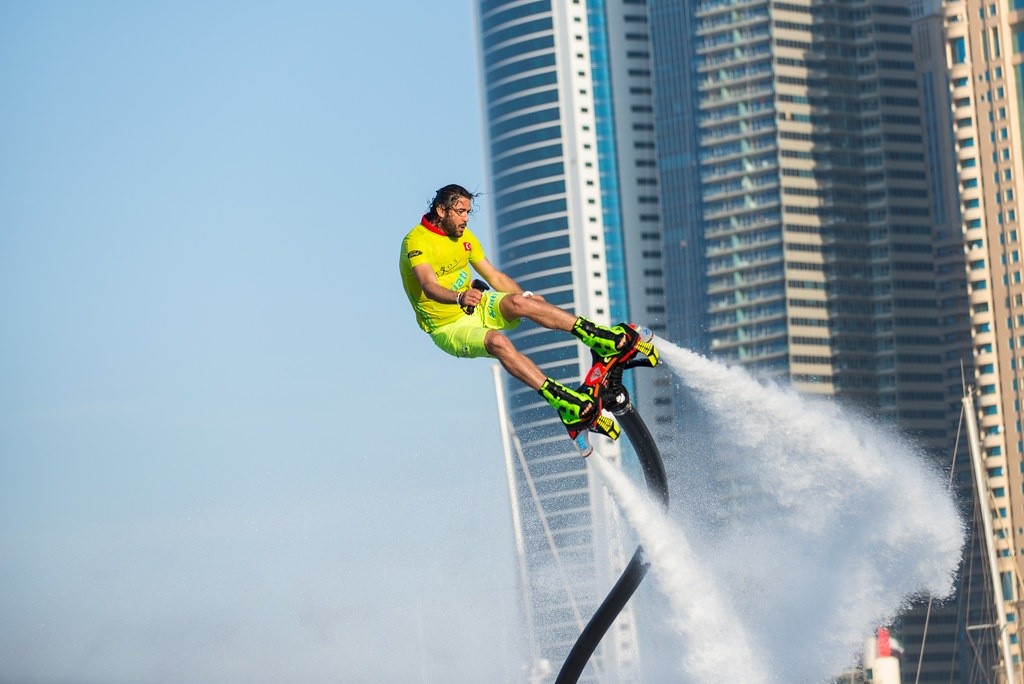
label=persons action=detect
[400,184,630,419]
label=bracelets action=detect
[457,291,462,303]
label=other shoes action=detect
[537,376,597,424]
[572,314,633,358]
